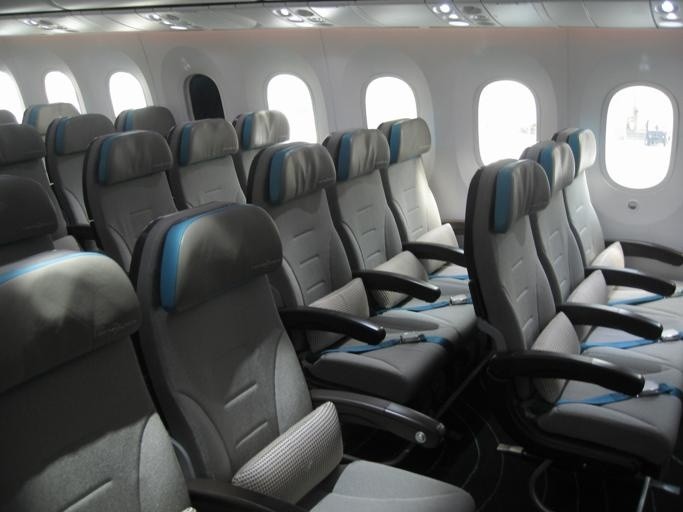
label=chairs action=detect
[0,103,682,512]
